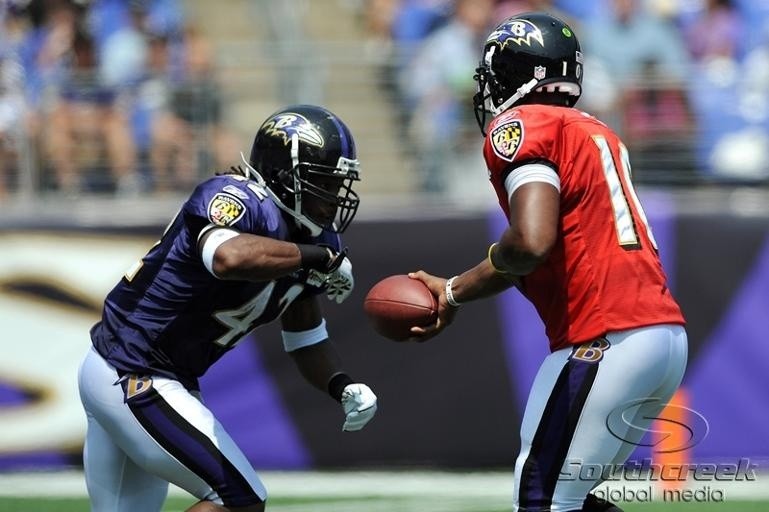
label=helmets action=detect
[471,10,585,119]
[246,106,362,238]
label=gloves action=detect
[319,248,355,304]
[338,381,380,434]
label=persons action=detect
[404,11,692,512]
[368,1,769,207]
[77,102,381,511]
[0,1,244,205]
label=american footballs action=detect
[363,274,438,341]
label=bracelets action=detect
[446,276,462,308]
[488,242,510,275]
[328,371,353,402]
[296,240,330,284]
